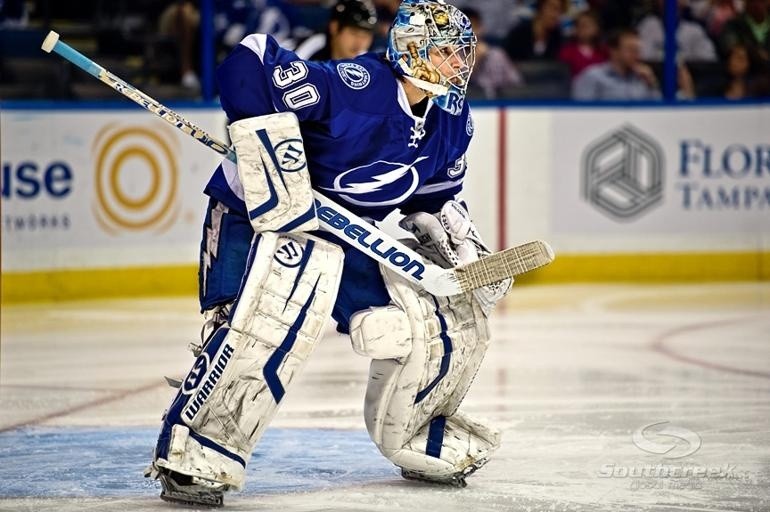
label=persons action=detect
[0,0,770,107]
[146,0,518,508]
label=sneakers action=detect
[162,467,223,498]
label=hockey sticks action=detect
[41,31,555,298]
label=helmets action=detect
[388,2,478,98]
[330,0,379,31]
